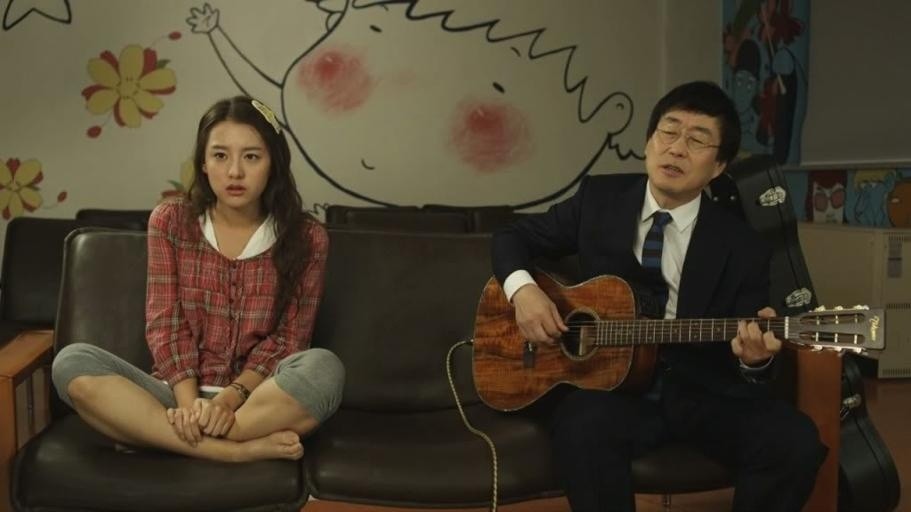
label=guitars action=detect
[471,262,888,421]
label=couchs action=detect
[0,221,846,512]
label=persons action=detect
[50,95,346,461]
[491,80,821,511]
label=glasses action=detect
[656,125,720,152]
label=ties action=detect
[642,211,672,319]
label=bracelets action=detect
[229,381,251,402]
